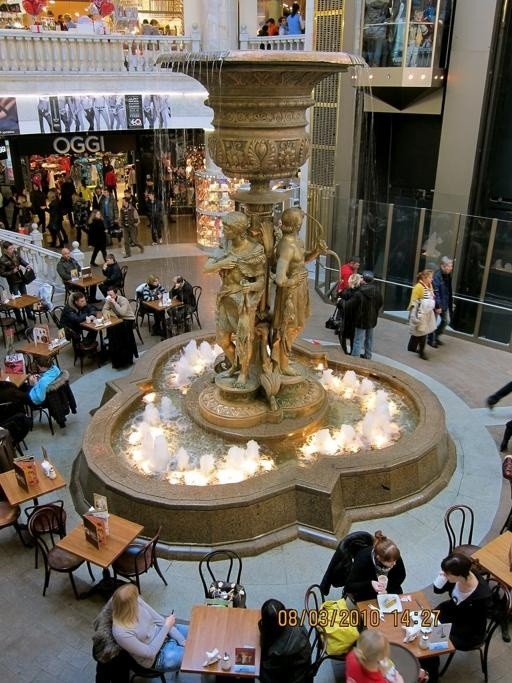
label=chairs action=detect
[304,505,511,682]
[130,662,167,683]
[199,549,242,598]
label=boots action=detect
[48,237,67,248]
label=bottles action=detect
[419,635,429,649]
[48,344,53,352]
[48,468,56,479]
[221,652,231,670]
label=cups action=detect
[378,575,388,589]
[105,296,111,302]
[434,575,448,589]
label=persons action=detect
[274,208,328,374]
[258,599,311,683]
[332,246,461,366]
[141,189,167,246]
[25,354,60,413]
[136,271,194,336]
[7,151,145,259]
[202,212,267,385]
[344,628,404,683]
[256,3,305,49]
[34,95,173,134]
[64,290,136,368]
[95,253,125,298]
[0,378,34,448]
[417,552,493,683]
[57,249,84,288]
[110,582,190,673]
[0,1,184,51]
[334,531,407,603]
[2,239,33,299]
[486,380,512,452]
[363,1,431,67]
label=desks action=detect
[180,605,262,683]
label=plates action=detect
[411,610,440,626]
[377,593,403,613]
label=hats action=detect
[360,271,374,282]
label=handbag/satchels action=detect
[324,318,340,330]
[23,267,35,284]
[210,581,248,606]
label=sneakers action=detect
[410,349,426,360]
[360,353,367,358]
[124,246,145,258]
[91,263,98,266]
[151,238,163,245]
[427,340,443,347]
